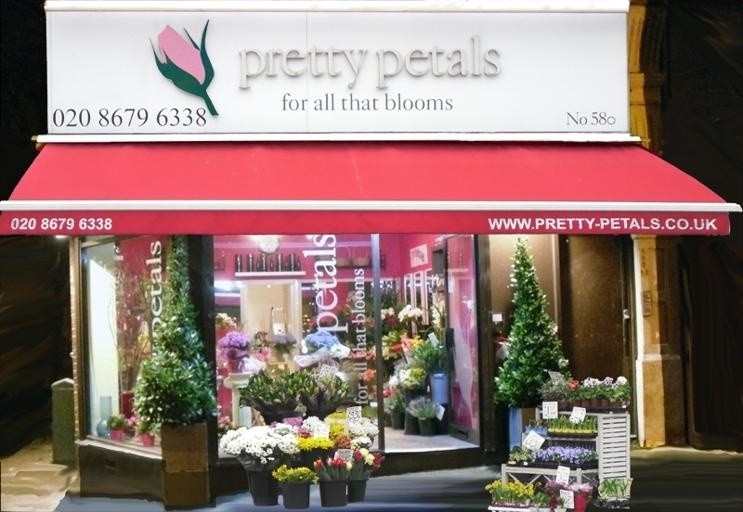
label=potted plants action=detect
[495,232,570,453]
[106,234,218,512]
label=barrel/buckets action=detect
[429,373,450,405]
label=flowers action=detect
[216,323,387,483]
[360,300,452,422]
[485,370,636,512]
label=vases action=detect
[247,461,368,510]
[95,394,116,438]
[390,406,444,436]
